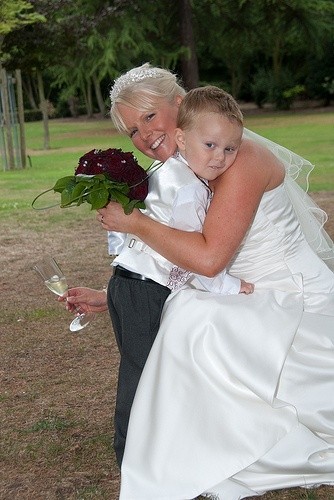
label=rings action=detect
[101,215,104,224]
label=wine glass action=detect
[32,256,95,332]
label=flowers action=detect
[74,148,149,202]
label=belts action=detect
[112,266,153,282]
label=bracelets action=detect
[99,285,108,295]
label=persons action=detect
[106,85,254,471]
[55,63,334,500]
